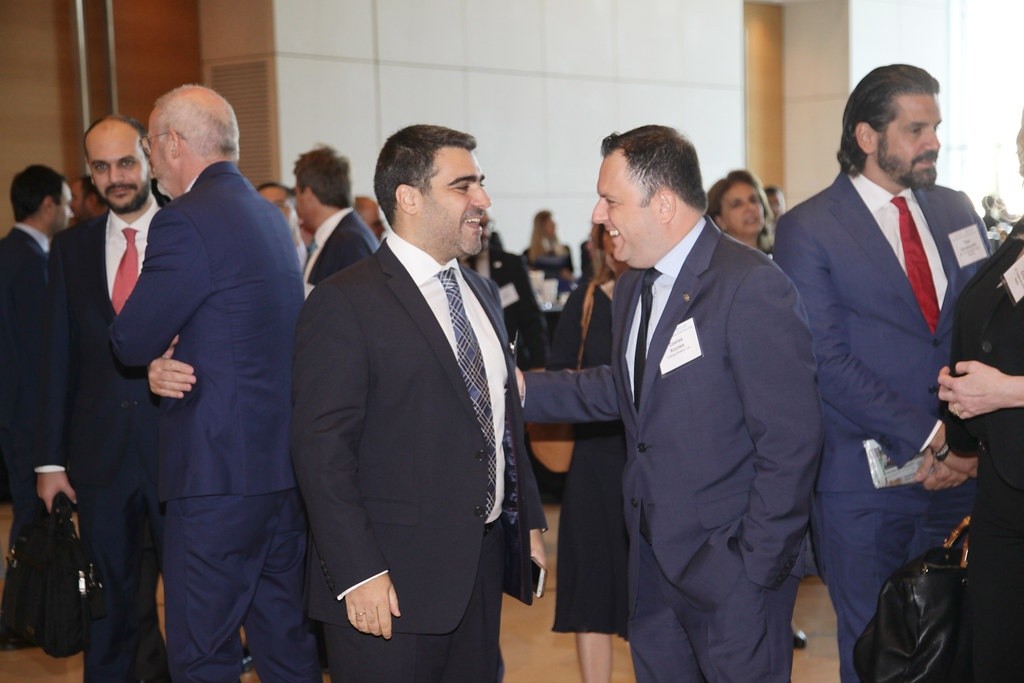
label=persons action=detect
[774,65,995,683]
[104,82,323,683]
[938,110,1024,683]
[0,165,78,648]
[514,125,823,683]
[78,174,1020,648]
[297,147,381,678]
[552,221,630,683]
[31,113,173,683]
[239,183,307,675]
[291,123,548,683]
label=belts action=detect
[483,514,502,535]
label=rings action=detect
[356,613,365,615]
[951,407,960,417]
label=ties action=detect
[634,267,662,415]
[435,267,496,520]
[111,228,139,315]
[891,196,940,336]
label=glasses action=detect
[140,131,186,156]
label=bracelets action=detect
[936,447,951,461]
[931,440,946,457]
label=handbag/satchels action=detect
[853,514,970,683]
[525,423,575,474]
[2,497,90,657]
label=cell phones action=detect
[530,557,547,598]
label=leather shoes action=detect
[0,625,33,650]
